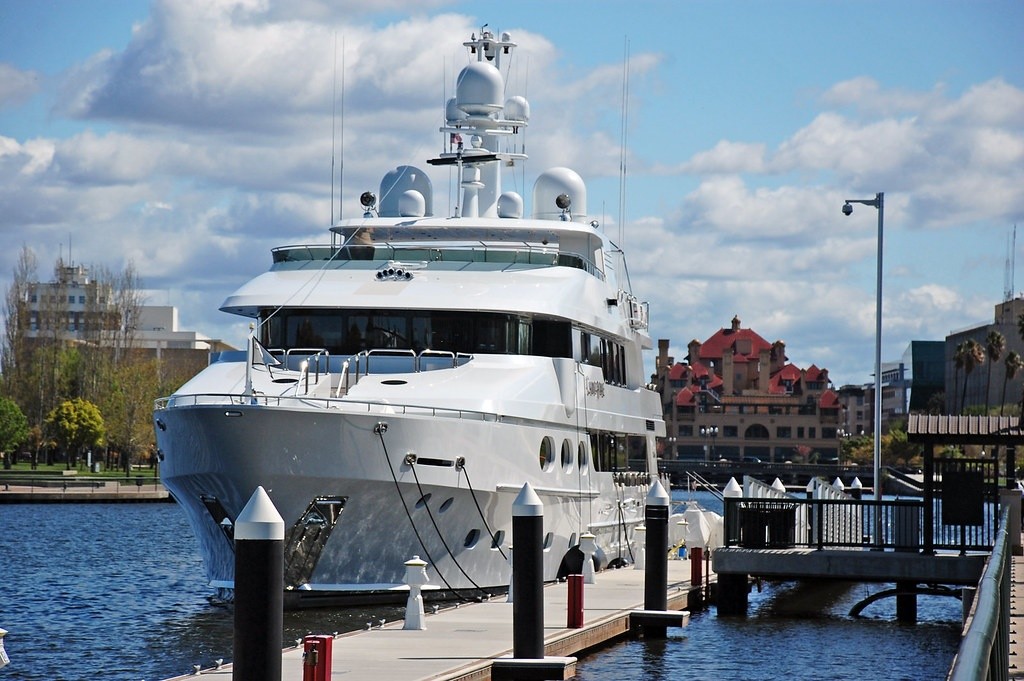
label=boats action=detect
[147,21,671,613]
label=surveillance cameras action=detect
[841,205,852,216]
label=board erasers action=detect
[893,498,920,553]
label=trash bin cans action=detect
[742,503,799,551]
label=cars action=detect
[742,456,772,469]
[894,466,922,475]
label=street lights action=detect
[668,436,676,460]
[836,429,845,465]
[701,427,718,461]
[842,191,884,552]
